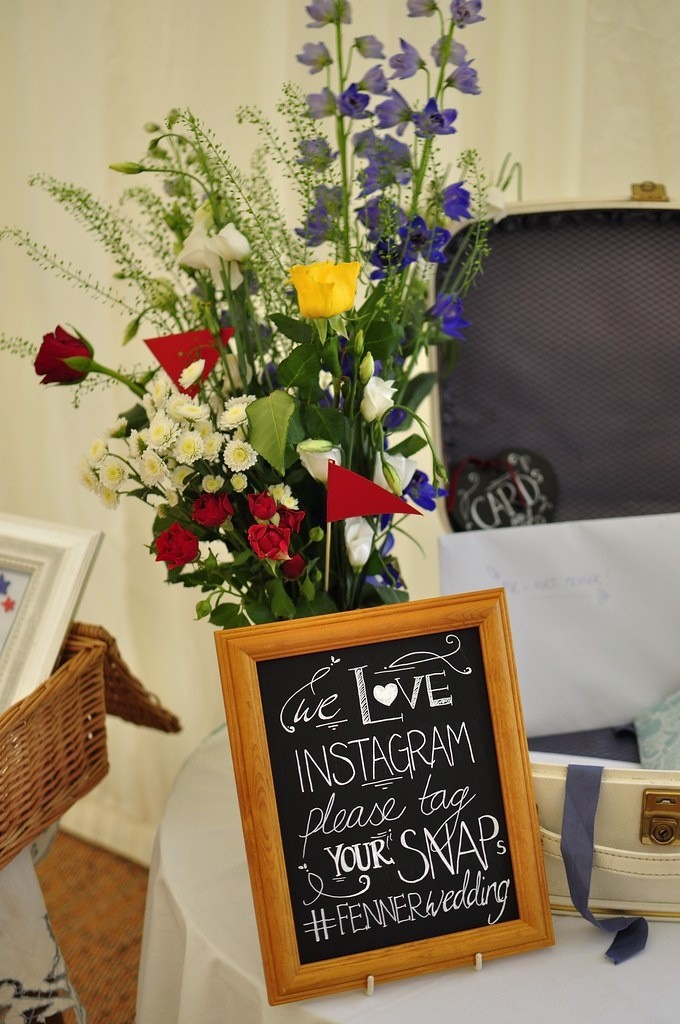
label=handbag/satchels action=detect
[527,761,680,922]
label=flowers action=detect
[0,0,498,631]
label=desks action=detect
[136,716,680,1024]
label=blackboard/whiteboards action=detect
[208,585,554,1007]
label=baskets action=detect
[0,623,181,867]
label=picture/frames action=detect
[213,586,554,1007]
[0,510,105,713]
